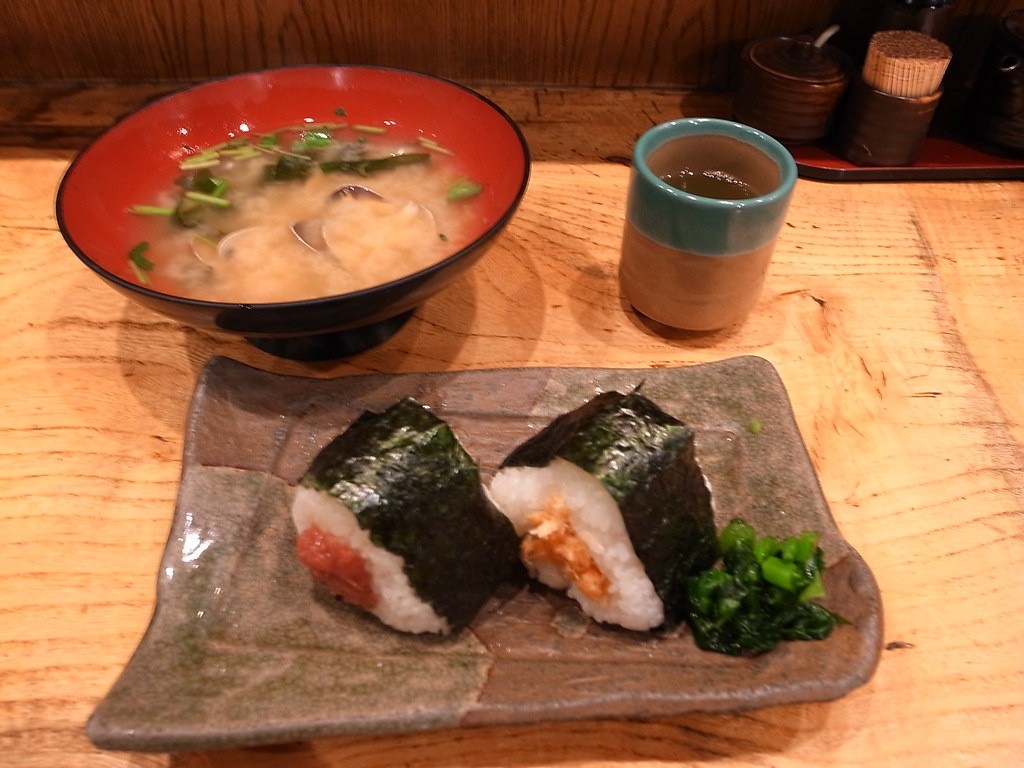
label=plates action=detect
[86,354,882,750]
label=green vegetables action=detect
[673,519,858,660]
[121,105,485,288]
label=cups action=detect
[618,119,799,333]
[835,72,944,168]
[733,34,854,144]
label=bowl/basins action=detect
[55,64,533,362]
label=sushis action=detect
[286,396,524,640]
[482,377,724,640]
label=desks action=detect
[0,159,1024,768]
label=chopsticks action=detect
[863,28,953,98]
